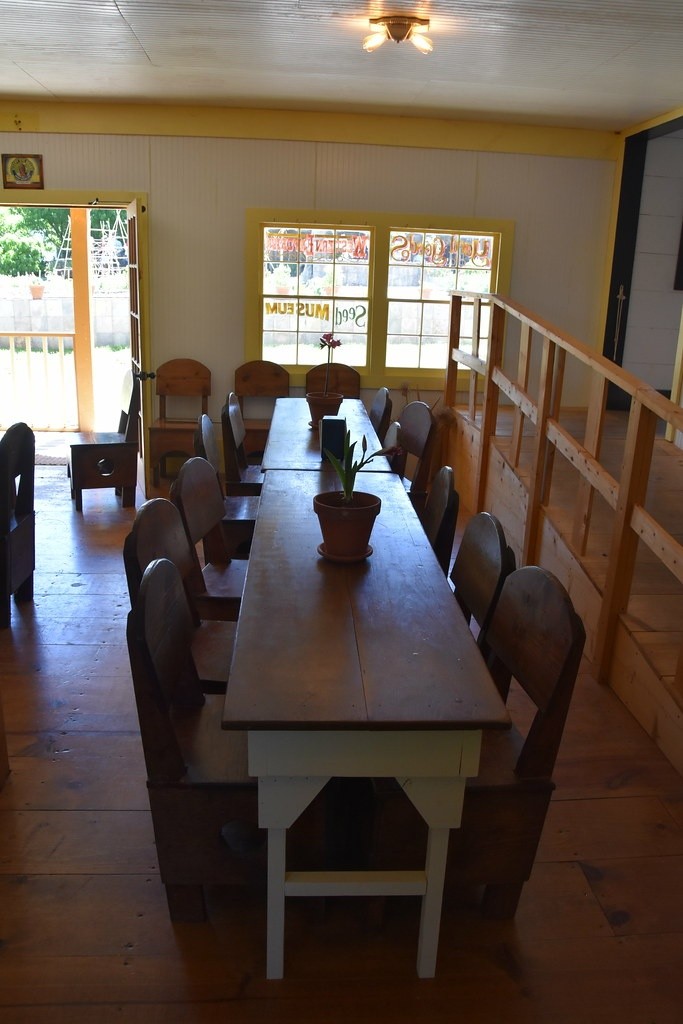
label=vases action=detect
[31,284,45,300]
[306,392,344,425]
[313,491,381,556]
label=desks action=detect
[260,398,392,473]
[220,470,513,979]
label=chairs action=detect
[149,358,211,488]
[235,360,290,432]
[123,386,587,922]
[306,364,361,399]
[0,421,36,629]
[66,371,141,512]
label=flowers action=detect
[322,430,402,503]
[320,333,341,398]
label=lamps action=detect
[362,15,434,56]
